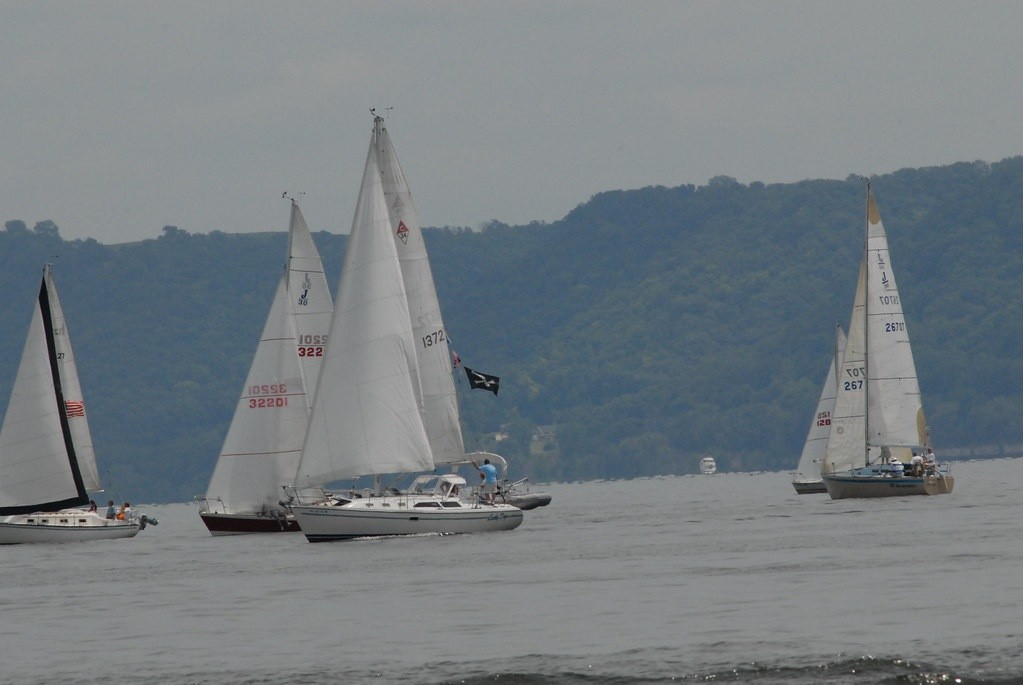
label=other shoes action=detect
[491,502,494,506]
[487,500,491,504]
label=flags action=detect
[464,365,500,396]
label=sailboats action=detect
[793,324,937,495]
[201,187,342,537]
[821,181,956,500]
[273,106,526,542]
[0,262,142,545]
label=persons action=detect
[88,499,132,521]
[889,456,905,478]
[910,447,936,478]
[471,459,498,506]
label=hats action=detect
[928,447,932,452]
[480,472,484,478]
[892,458,897,462]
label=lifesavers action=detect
[913,461,925,477]
